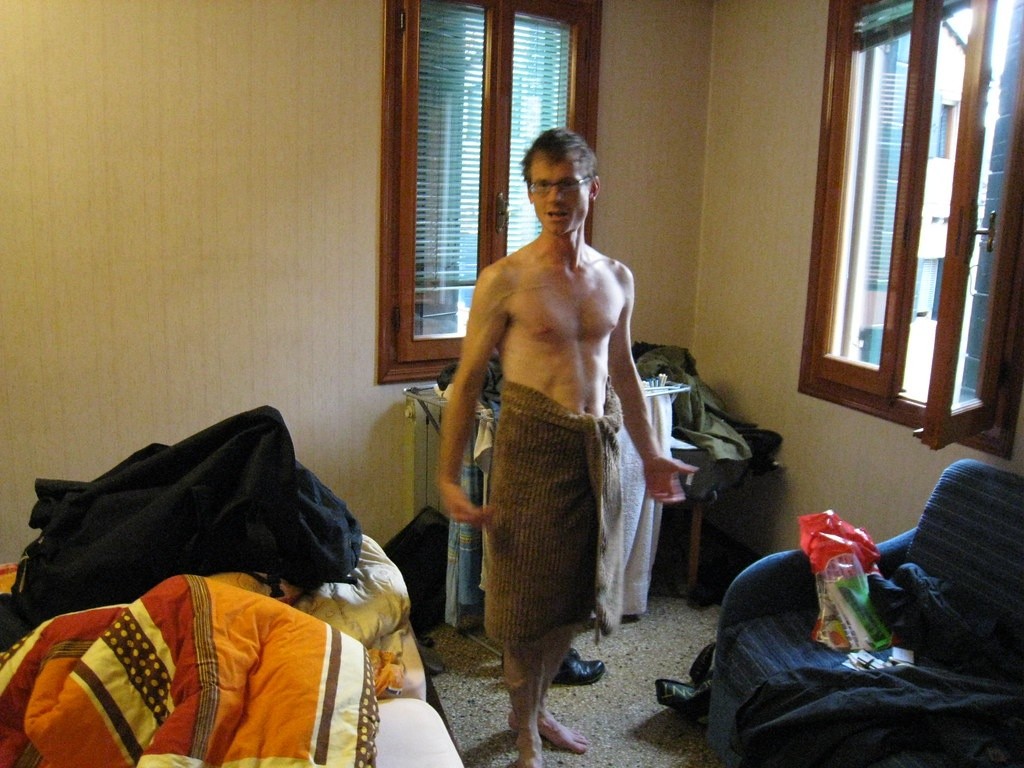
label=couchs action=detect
[706,460,1024,768]
[376,620,469,768]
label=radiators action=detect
[402,395,451,525]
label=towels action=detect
[619,382,674,618]
[481,377,628,650]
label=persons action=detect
[435,126,697,768]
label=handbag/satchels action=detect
[800,510,899,650]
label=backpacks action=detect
[381,507,449,646]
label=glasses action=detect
[528,177,592,191]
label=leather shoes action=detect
[501,647,577,667]
[553,657,605,683]
